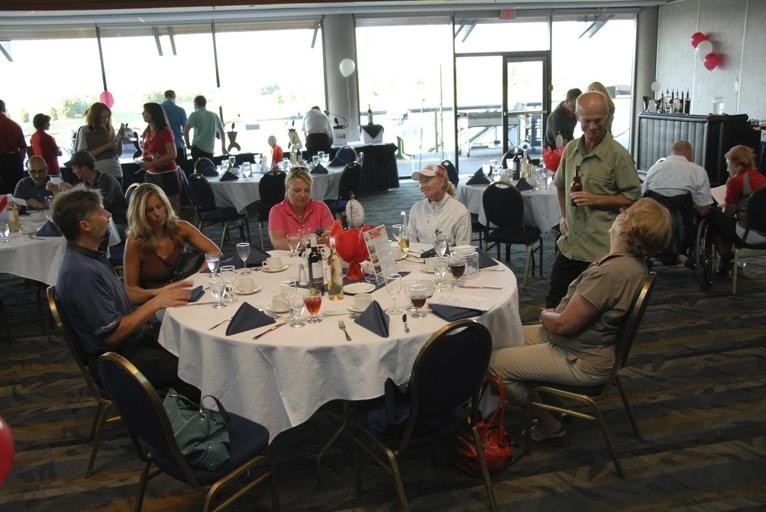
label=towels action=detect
[427,301,490,326]
[352,300,394,339]
[225,302,275,337]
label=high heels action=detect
[519,406,575,443]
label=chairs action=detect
[95,350,285,510]
[504,270,661,482]
[340,314,496,511]
[42,283,173,475]
[713,184,764,294]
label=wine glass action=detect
[214,152,330,177]
[383,222,467,317]
[203,230,322,328]
[0,194,56,244]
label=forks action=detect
[337,319,352,341]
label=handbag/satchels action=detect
[450,372,516,475]
[27,145,33,158]
[151,385,231,471]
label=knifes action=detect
[253,322,286,338]
[402,313,409,334]
[459,285,502,290]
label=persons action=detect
[709,145,766,280]
[406,163,471,247]
[121,177,222,303]
[486,196,673,441]
[543,92,643,308]
[51,188,201,406]
[543,88,581,151]
[642,137,714,267]
[265,168,335,252]
[0,89,231,223]
[587,81,615,139]
[301,106,334,162]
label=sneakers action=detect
[714,249,743,279]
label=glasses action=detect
[26,167,48,175]
[289,166,310,174]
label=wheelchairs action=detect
[640,190,715,291]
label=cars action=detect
[19,118,147,170]
[179,127,194,161]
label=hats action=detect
[410,161,448,183]
[63,149,96,169]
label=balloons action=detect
[688,28,720,74]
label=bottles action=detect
[712,96,726,114]
[398,209,411,251]
[658,87,692,115]
[571,164,583,206]
[511,147,531,181]
[325,238,345,300]
[367,104,373,125]
[450,245,480,280]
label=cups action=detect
[353,294,372,310]
[482,159,551,190]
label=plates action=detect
[344,282,376,293]
[345,305,366,313]
